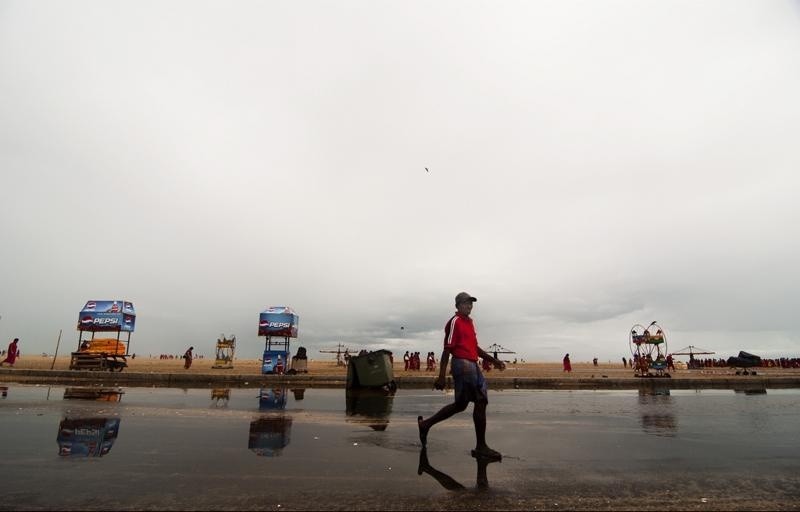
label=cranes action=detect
[672,345,717,362]
[318,342,359,365]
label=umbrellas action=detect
[672,344,716,359]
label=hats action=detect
[456,292,476,305]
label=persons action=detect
[0,385,10,399]
[667,353,677,372]
[622,328,666,376]
[685,355,800,369]
[562,352,572,373]
[403,349,437,372]
[593,357,599,368]
[0,337,19,367]
[184,346,194,370]
[416,459,498,494]
[417,291,507,462]
[80,340,91,351]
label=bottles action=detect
[264,352,283,373]
[111,302,118,314]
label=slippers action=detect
[472,449,501,460]
[418,415,428,444]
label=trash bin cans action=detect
[353,349,396,391]
